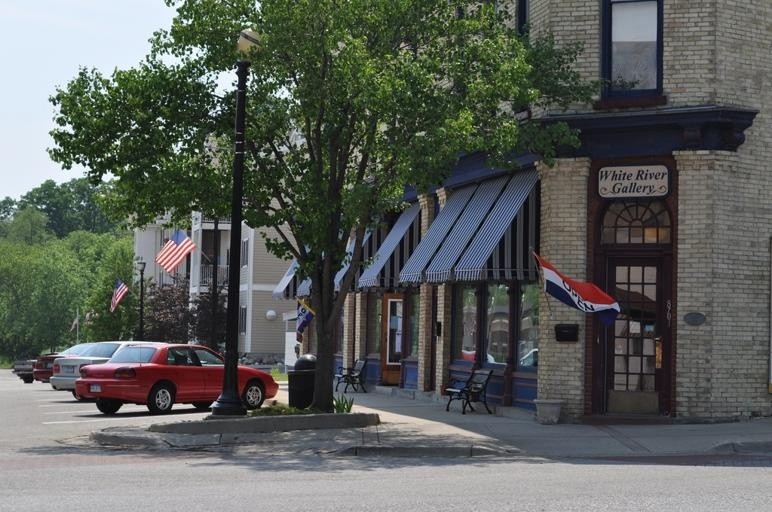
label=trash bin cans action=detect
[288,354,317,410]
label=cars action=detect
[11,341,279,415]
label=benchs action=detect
[334,359,367,393]
[444,369,493,414]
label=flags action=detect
[292,297,317,362]
[110,276,132,315]
[538,260,620,316]
[154,229,198,274]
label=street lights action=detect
[137,257,146,340]
[208,28,261,415]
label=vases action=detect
[533,399,567,425]
